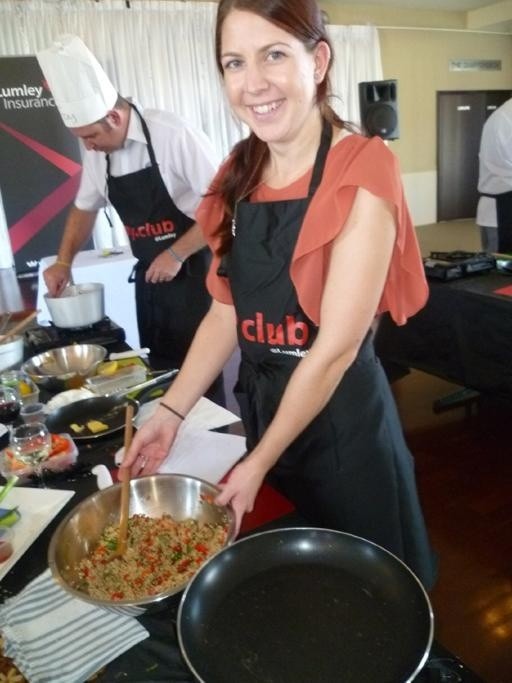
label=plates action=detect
[0,485,76,581]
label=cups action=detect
[20,403,46,424]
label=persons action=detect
[110,0,442,596]
[45,91,226,424]
[471,94,512,255]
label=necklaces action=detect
[230,134,320,238]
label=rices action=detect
[66,515,229,602]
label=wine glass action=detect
[0,388,22,442]
[10,422,51,489]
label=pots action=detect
[44,282,104,329]
[177,527,435,683]
[50,369,179,440]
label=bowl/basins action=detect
[0,335,24,372]
[21,344,107,393]
[48,473,236,618]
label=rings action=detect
[140,455,145,458]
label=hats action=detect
[37,33,120,130]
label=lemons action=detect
[97,361,116,376]
[19,380,31,394]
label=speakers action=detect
[358,79,401,141]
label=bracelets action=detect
[55,257,72,272]
[166,244,188,265]
[157,400,187,424]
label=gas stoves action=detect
[423,250,497,279]
[25,314,126,357]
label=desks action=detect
[38,246,141,351]
[374,267,512,399]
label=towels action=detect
[136,393,242,431]
[1,564,154,682]
[154,430,246,484]
